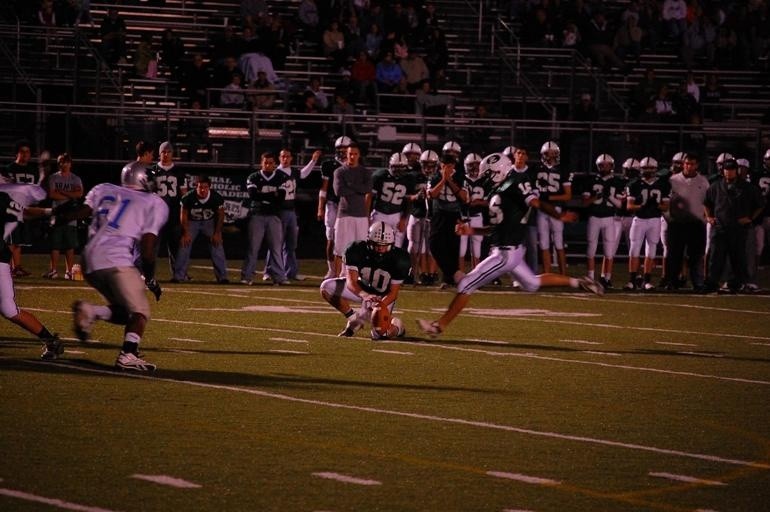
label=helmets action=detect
[389,141,482,178]
[367,221,395,256]
[121,161,158,192]
[503,145,517,157]
[540,140,560,169]
[596,149,770,196]
[335,135,353,161]
[478,153,517,200]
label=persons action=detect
[415,154,605,339]
[320,222,412,340]
[1,1,769,291]
[0,168,65,361]
[71,160,169,373]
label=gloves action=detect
[51,196,87,216]
[145,277,162,302]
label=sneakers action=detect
[11,265,73,280]
[402,272,439,286]
[338,313,364,337]
[391,318,405,337]
[417,319,442,339]
[115,350,157,372]
[71,299,95,342]
[606,277,770,294]
[41,338,63,360]
[579,276,605,298]
[239,273,305,287]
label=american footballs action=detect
[372,301,390,337]
